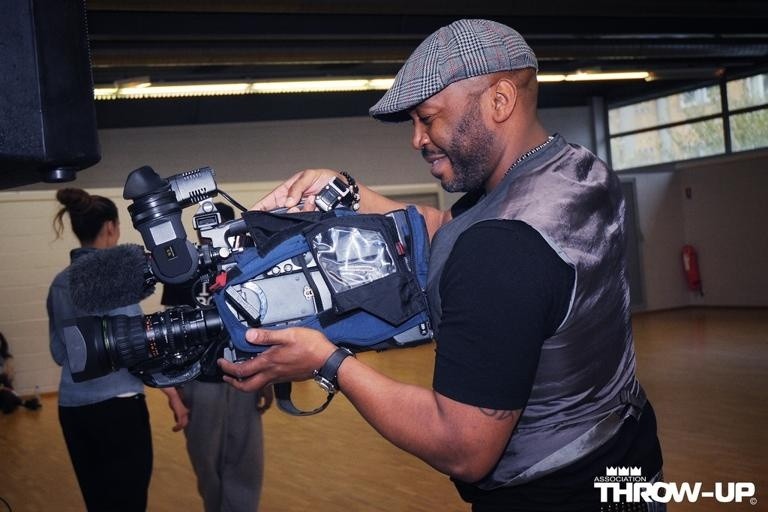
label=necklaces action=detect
[503,136,554,177]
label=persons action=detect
[217,20,669,511]
[44,185,190,511]
[161,203,273,512]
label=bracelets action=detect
[310,348,355,395]
[343,167,360,212]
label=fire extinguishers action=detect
[680,243,703,294]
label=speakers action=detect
[0,0,102,193]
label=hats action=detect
[368,19,538,122]
